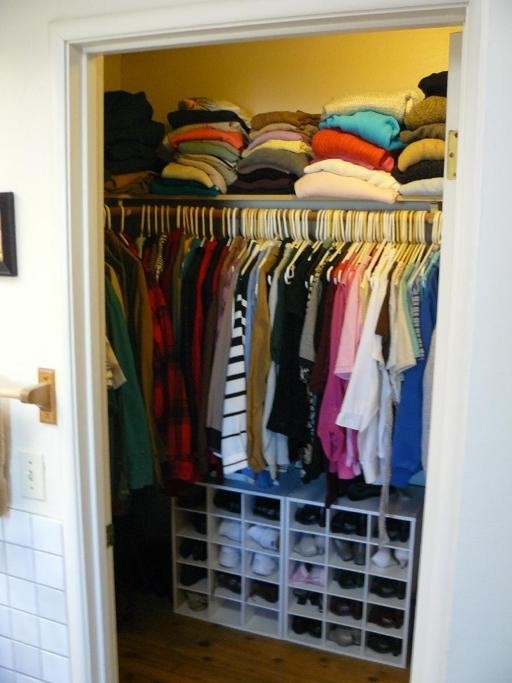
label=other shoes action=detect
[176,488,411,656]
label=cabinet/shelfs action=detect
[166,474,426,669]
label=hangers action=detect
[103,196,440,288]
